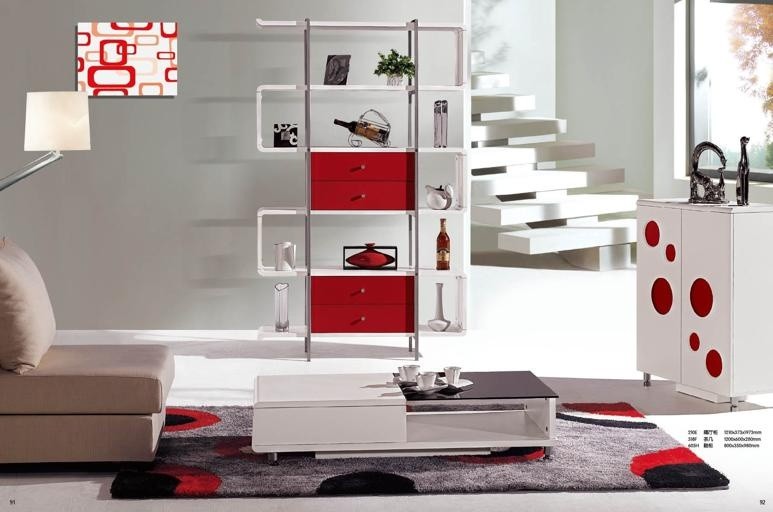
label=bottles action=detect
[331,119,391,143]
[734,136,750,206]
[434,217,450,269]
[346,242,394,268]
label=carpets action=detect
[110,398,731,503]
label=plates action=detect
[392,374,474,394]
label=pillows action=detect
[0,235,58,373]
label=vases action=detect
[427,281,451,332]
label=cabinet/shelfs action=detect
[633,195,773,406]
[251,16,469,344]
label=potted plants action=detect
[373,48,415,86]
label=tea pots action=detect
[423,184,454,212]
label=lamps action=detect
[0,88,91,196]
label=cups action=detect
[397,363,461,386]
[274,242,296,272]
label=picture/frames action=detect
[271,120,300,149]
[323,53,350,87]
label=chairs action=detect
[0,234,176,463]
